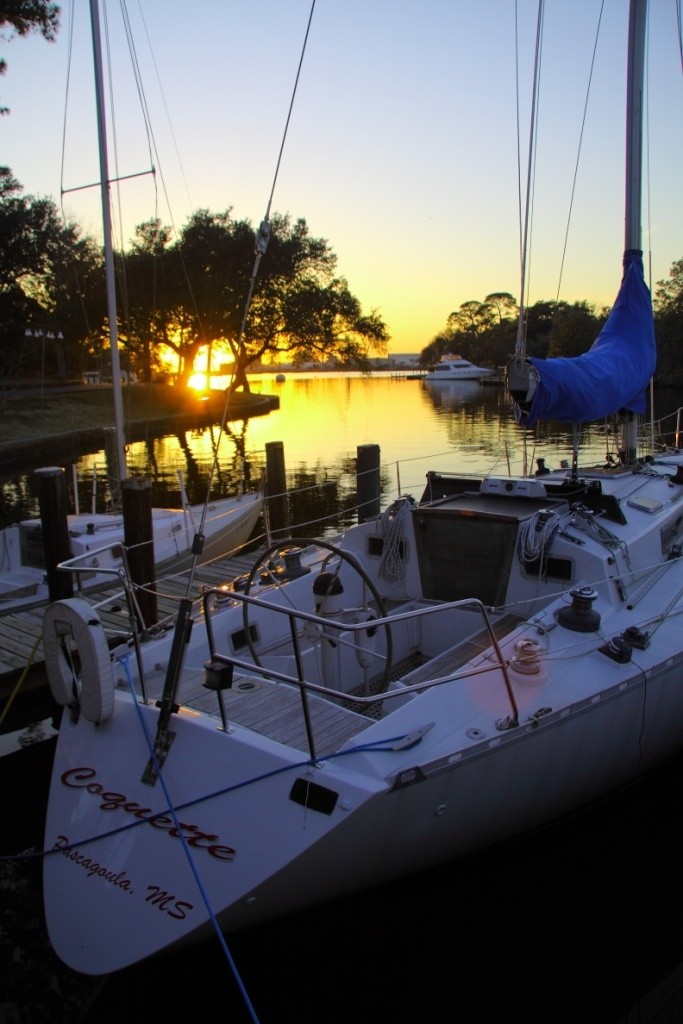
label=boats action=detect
[276,374,285,381]
[425,352,493,380]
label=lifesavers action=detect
[43,597,115,723]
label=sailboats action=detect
[0,0,683,978]
